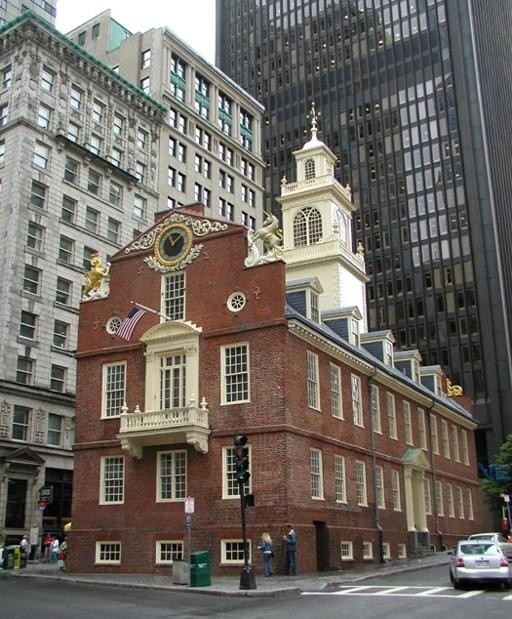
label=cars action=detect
[446,532,512,589]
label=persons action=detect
[43,534,54,563]
[255,532,275,579]
[59,536,68,568]
[50,536,60,563]
[19,533,30,569]
[281,524,298,577]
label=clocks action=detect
[154,224,193,266]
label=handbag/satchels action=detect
[57,552,64,560]
[270,552,275,558]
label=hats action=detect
[22,534,29,539]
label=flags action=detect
[114,306,145,342]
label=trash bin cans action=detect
[172,559,189,585]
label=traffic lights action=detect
[234,435,250,483]
[245,494,254,507]
